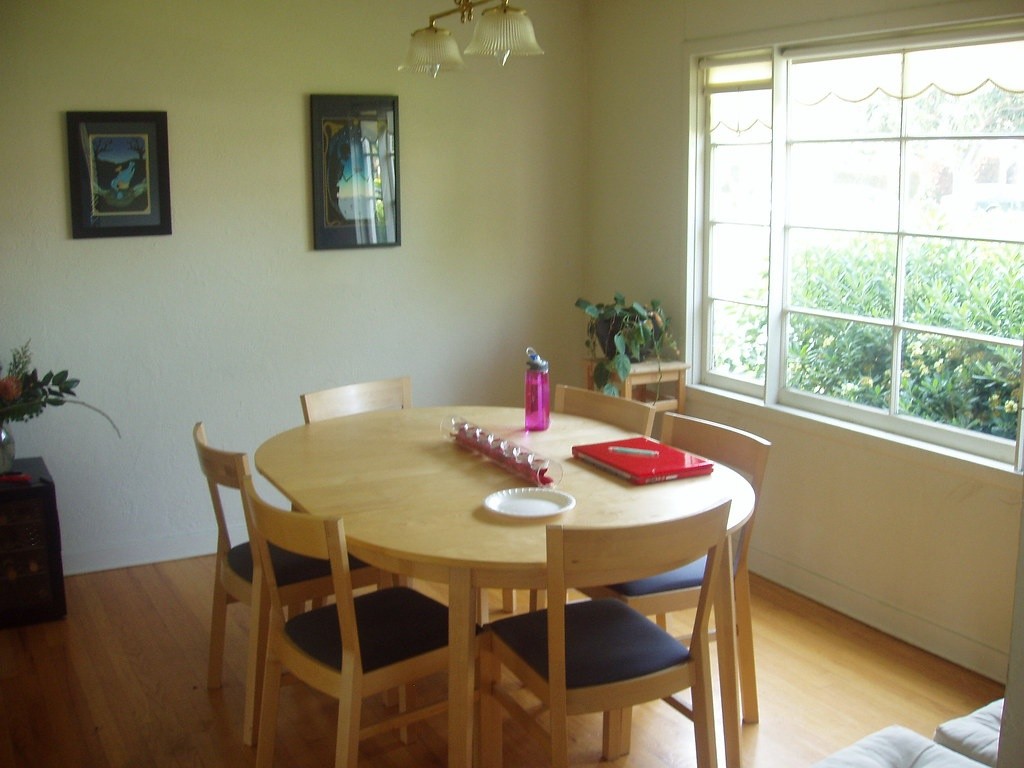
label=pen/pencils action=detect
[607,446,660,458]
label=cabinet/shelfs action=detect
[0,457,68,631]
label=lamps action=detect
[407,0,547,83]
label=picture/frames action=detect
[64,111,175,240]
[307,93,402,251]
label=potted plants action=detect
[572,291,682,404]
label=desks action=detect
[252,404,757,768]
[582,354,691,421]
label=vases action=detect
[0,425,16,477]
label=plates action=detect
[483,485,575,518]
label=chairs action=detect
[192,376,772,768]
[802,491,1024,768]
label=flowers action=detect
[0,345,84,422]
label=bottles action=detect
[526,346,550,431]
